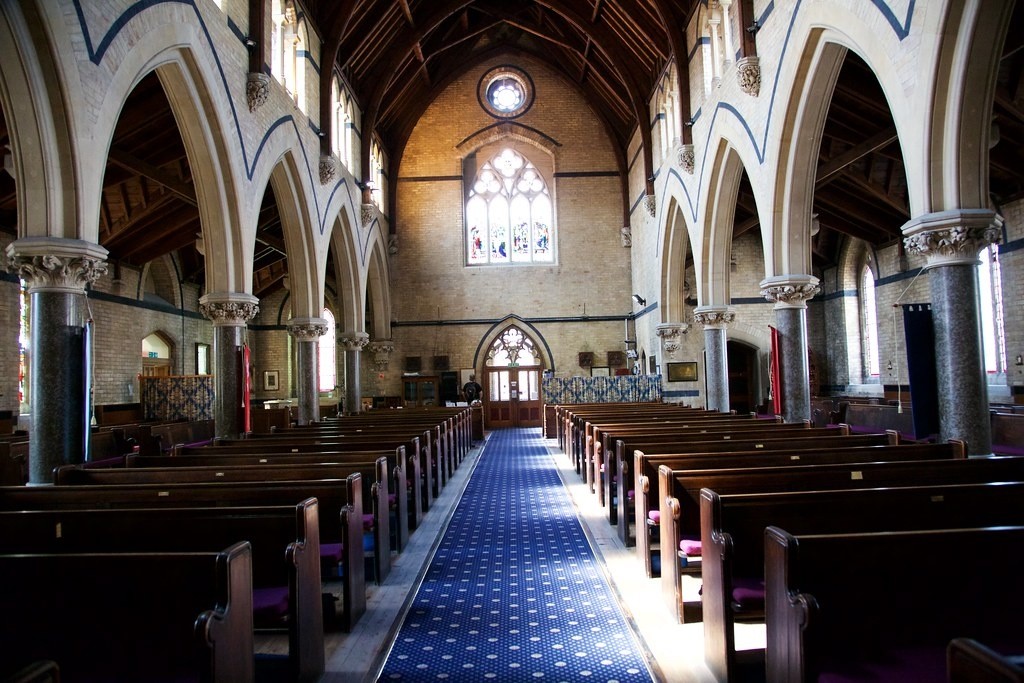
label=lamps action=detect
[632,295,646,306]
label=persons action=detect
[461,374,484,406]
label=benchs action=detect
[0,402,486,683]
[554,401,1024,683]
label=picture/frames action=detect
[667,362,698,382]
[263,370,279,391]
